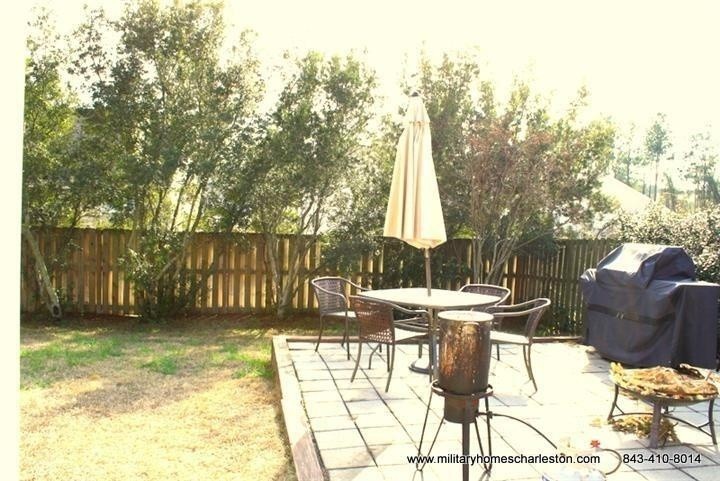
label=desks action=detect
[358,288,502,374]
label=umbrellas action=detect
[380,85,448,361]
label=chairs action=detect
[349,296,433,393]
[459,284,511,360]
[484,298,551,391]
[311,277,382,360]
